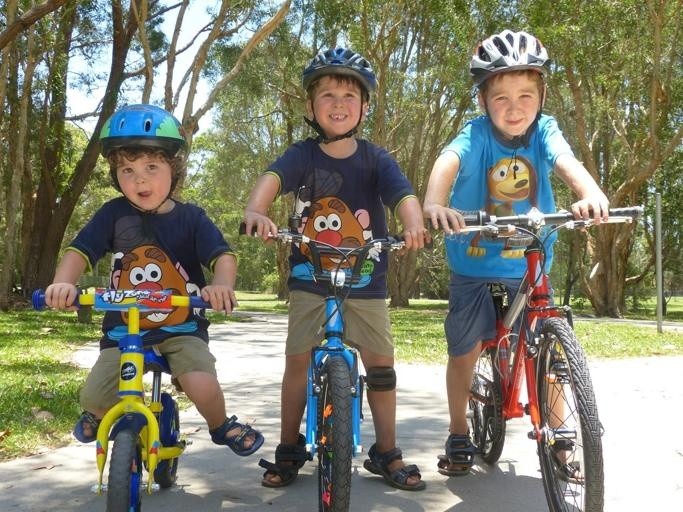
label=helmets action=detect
[302,48,376,91]
[99,104,186,153]
[470,29,548,84]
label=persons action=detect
[44,103,264,457]
[421,30,611,483]
[244,46,432,492]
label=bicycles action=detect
[432,207,644,511]
[237,221,434,511]
[32,284,234,511]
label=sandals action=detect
[209,415,305,488]
[537,440,585,484]
[75,411,99,442]
[363,444,470,491]
